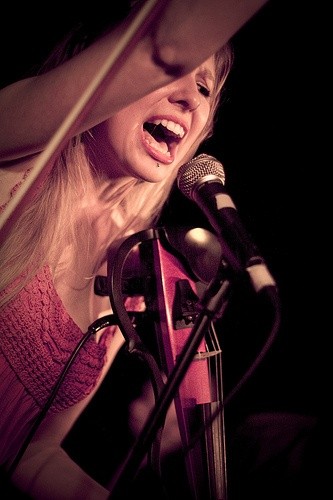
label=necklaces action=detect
[66,196,158,290]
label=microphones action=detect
[177,153,278,295]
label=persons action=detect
[0,0,266,500]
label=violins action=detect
[93,226,229,500]
